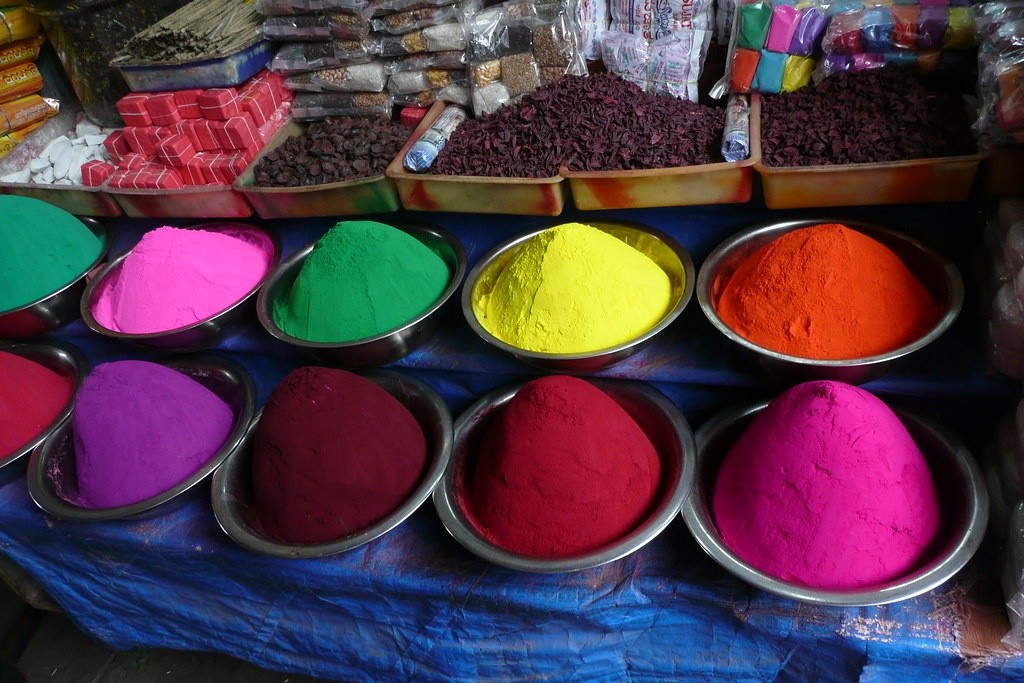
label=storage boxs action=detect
[982,148,1024,195]
[233,98,448,218]
[0,94,121,217]
[101,166,253,219]
[109,37,275,90]
[386,99,568,216]
[559,91,760,211]
[752,147,992,210]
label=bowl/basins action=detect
[0,338,89,487]
[256,222,468,366]
[80,220,283,354]
[432,377,698,572]
[697,217,965,385]
[681,397,989,606]
[212,366,454,559]
[0,216,114,340]
[462,218,695,372]
[27,354,257,521]
